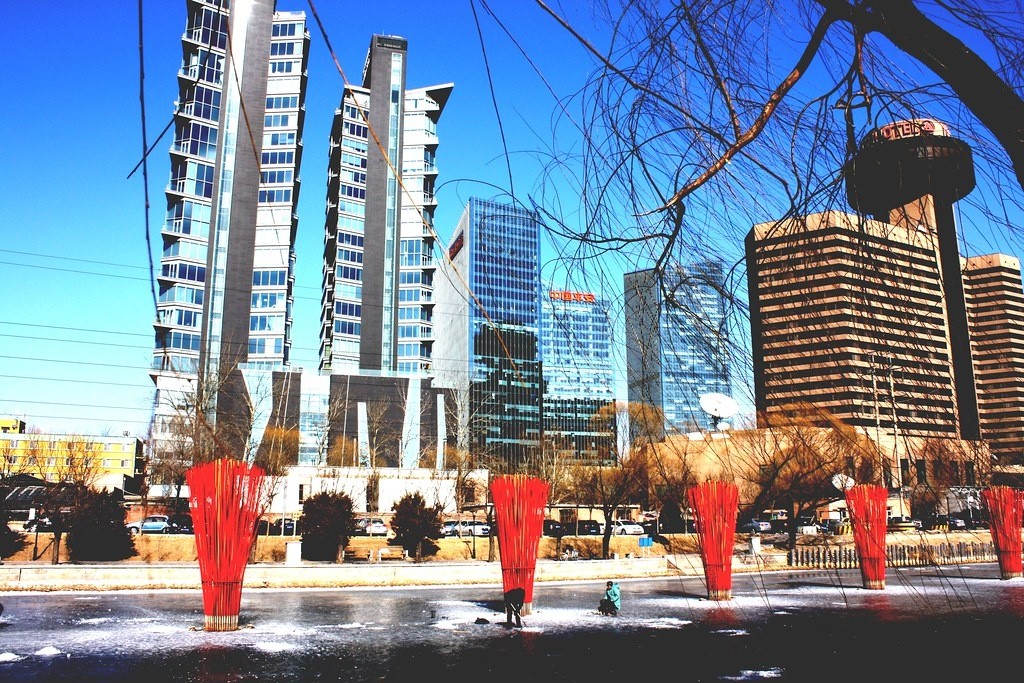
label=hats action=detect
[606,581,613,586]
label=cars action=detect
[22,513,995,538]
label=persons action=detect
[603,581,620,614]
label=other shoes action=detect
[615,612,619,616]
[606,613,612,616]
